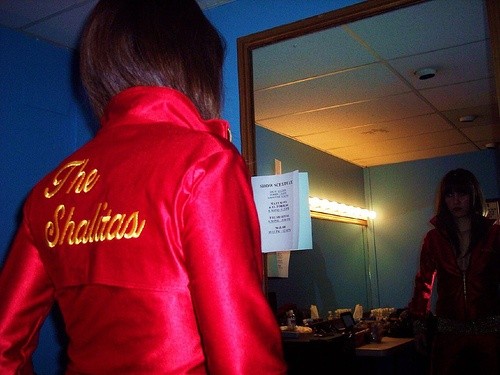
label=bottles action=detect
[328,311,333,319]
[337,308,352,314]
[286,309,296,329]
[334,311,338,319]
[371,308,396,319]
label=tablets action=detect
[340,311,356,330]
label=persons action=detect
[411,168,500,375]
[0,0,287,375]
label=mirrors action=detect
[236,0,500,375]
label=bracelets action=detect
[413,320,427,332]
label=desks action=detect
[354,336,415,375]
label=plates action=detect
[280,326,312,334]
